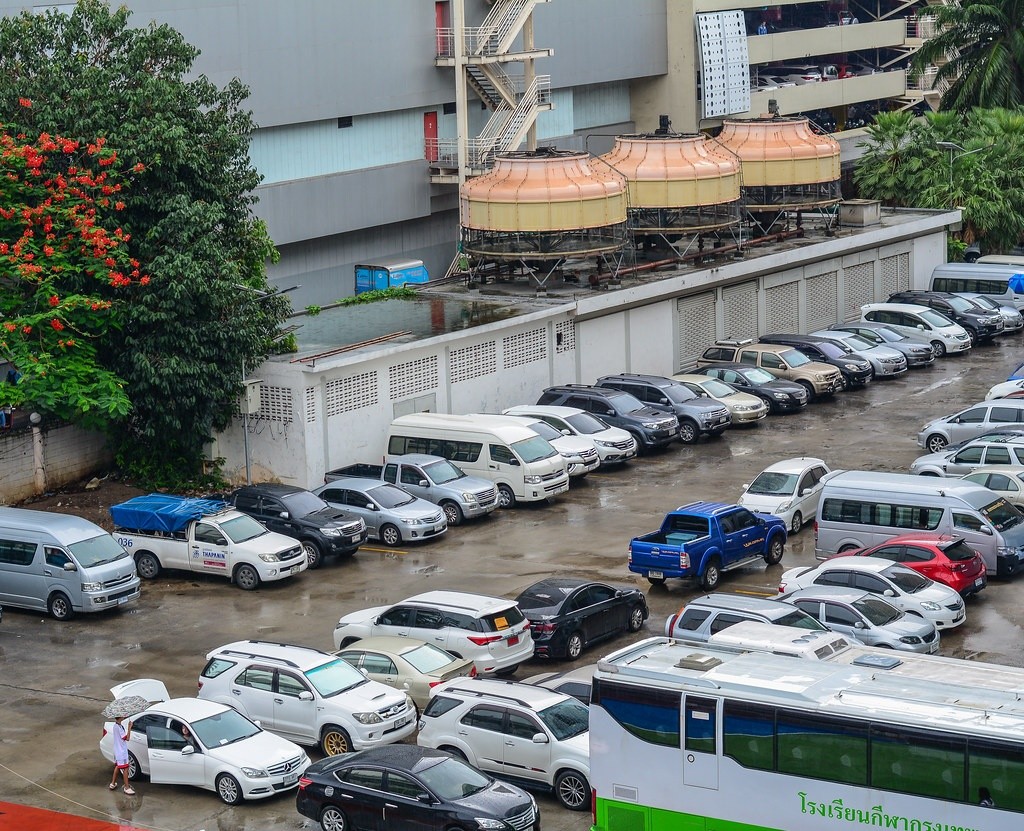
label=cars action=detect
[746,1,899,131]
[909,357,1024,513]
[965,245,981,261]
[520,662,598,701]
[737,456,831,534]
[514,576,652,662]
[768,588,941,654]
[327,636,478,711]
[296,751,542,830]
[99,681,313,806]
[764,531,987,654]
[807,290,1024,376]
[313,478,451,546]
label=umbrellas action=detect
[100,696,151,724]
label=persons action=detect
[757,21,768,35]
[844,105,856,130]
[108,717,136,795]
[177,724,199,752]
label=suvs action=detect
[418,675,590,813]
[468,334,874,478]
[666,594,866,648]
[196,639,420,758]
[226,481,368,565]
[335,588,537,675]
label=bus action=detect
[929,262,1024,314]
[586,619,1023,831]
[0,506,143,620]
[383,413,570,508]
[815,470,1024,577]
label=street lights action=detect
[937,141,996,241]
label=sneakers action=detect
[110,782,117,789]
[124,787,135,794]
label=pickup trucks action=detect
[628,498,789,588]
[111,496,310,588]
[324,451,501,527]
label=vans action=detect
[977,255,1024,267]
